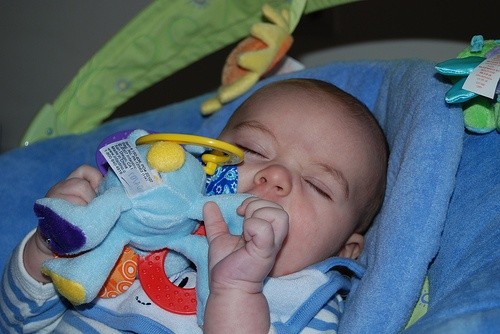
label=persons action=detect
[0,78,390,333]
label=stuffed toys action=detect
[197,2,296,116]
[34,129,258,331]
[432,34,500,136]
